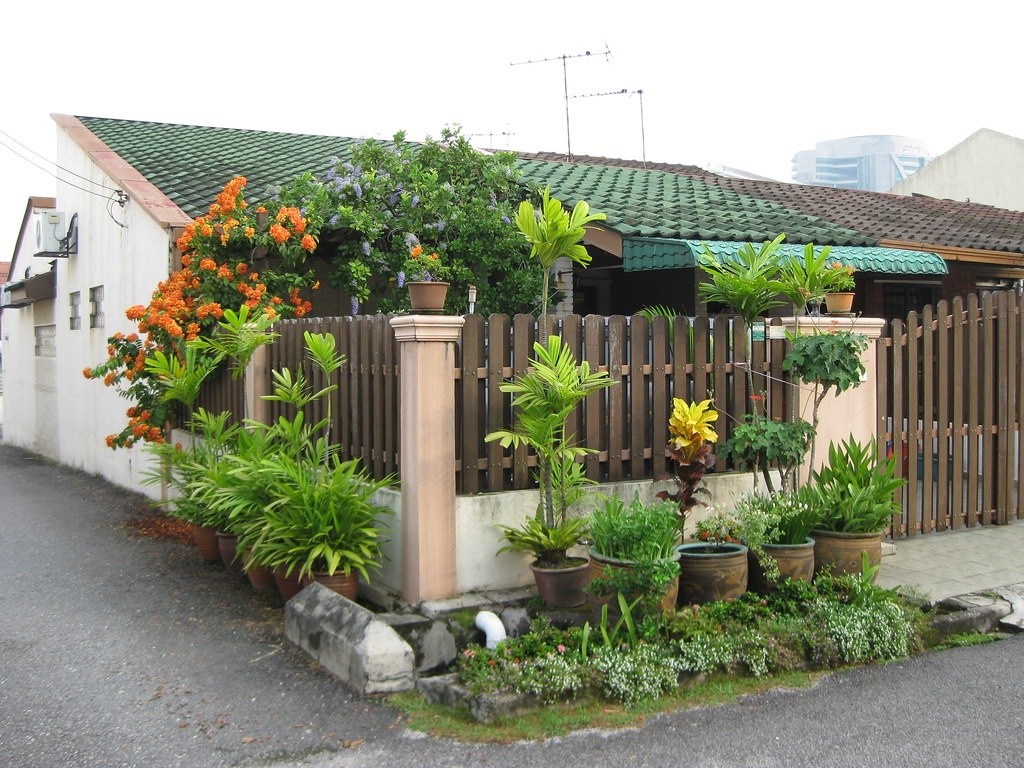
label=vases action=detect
[406,282,450,309]
[824,293,855,313]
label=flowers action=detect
[823,262,856,293]
[412,247,439,262]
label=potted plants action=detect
[484,182,908,621]
[143,305,400,602]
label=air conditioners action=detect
[34,211,66,254]
